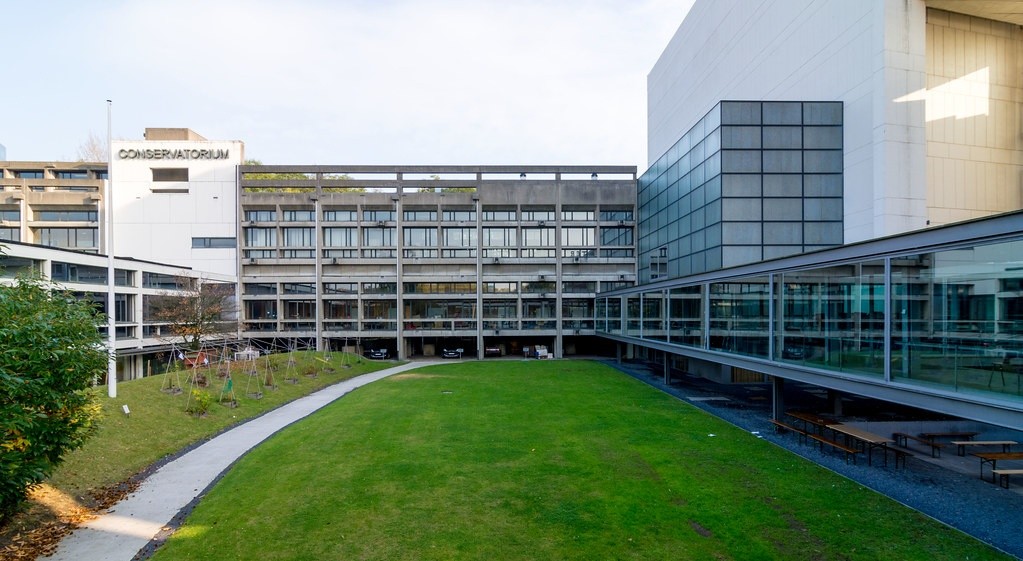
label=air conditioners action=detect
[538,221,546,227]
[332,257,340,263]
[618,220,626,227]
[538,275,547,282]
[617,274,627,282]
[573,257,581,264]
[377,220,385,226]
[249,257,258,265]
[493,257,500,264]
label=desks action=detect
[826,423,894,466]
[923,431,978,458]
[785,410,835,452]
[976,452,1023,480]
[951,436,1017,456]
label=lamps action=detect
[391,194,400,203]
[308,194,318,202]
[13,193,24,200]
[91,194,102,199]
[472,193,480,203]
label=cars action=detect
[484,341,502,358]
[440,340,461,359]
[367,340,391,360]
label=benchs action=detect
[895,432,946,458]
[991,469,1023,489]
[771,419,865,466]
